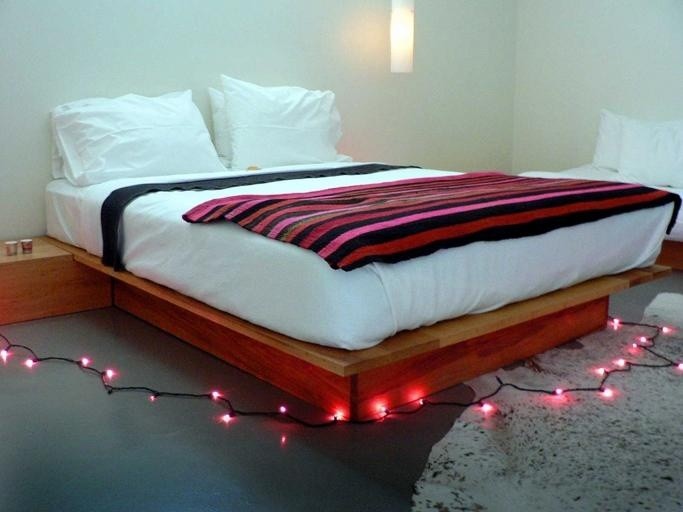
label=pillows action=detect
[51,91,227,189]
[205,74,350,172]
[593,107,683,188]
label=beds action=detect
[516,165,683,273]
[43,162,683,350]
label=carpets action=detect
[408,291,681,511]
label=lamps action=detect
[388,0,415,74]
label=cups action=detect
[4,239,32,256]
